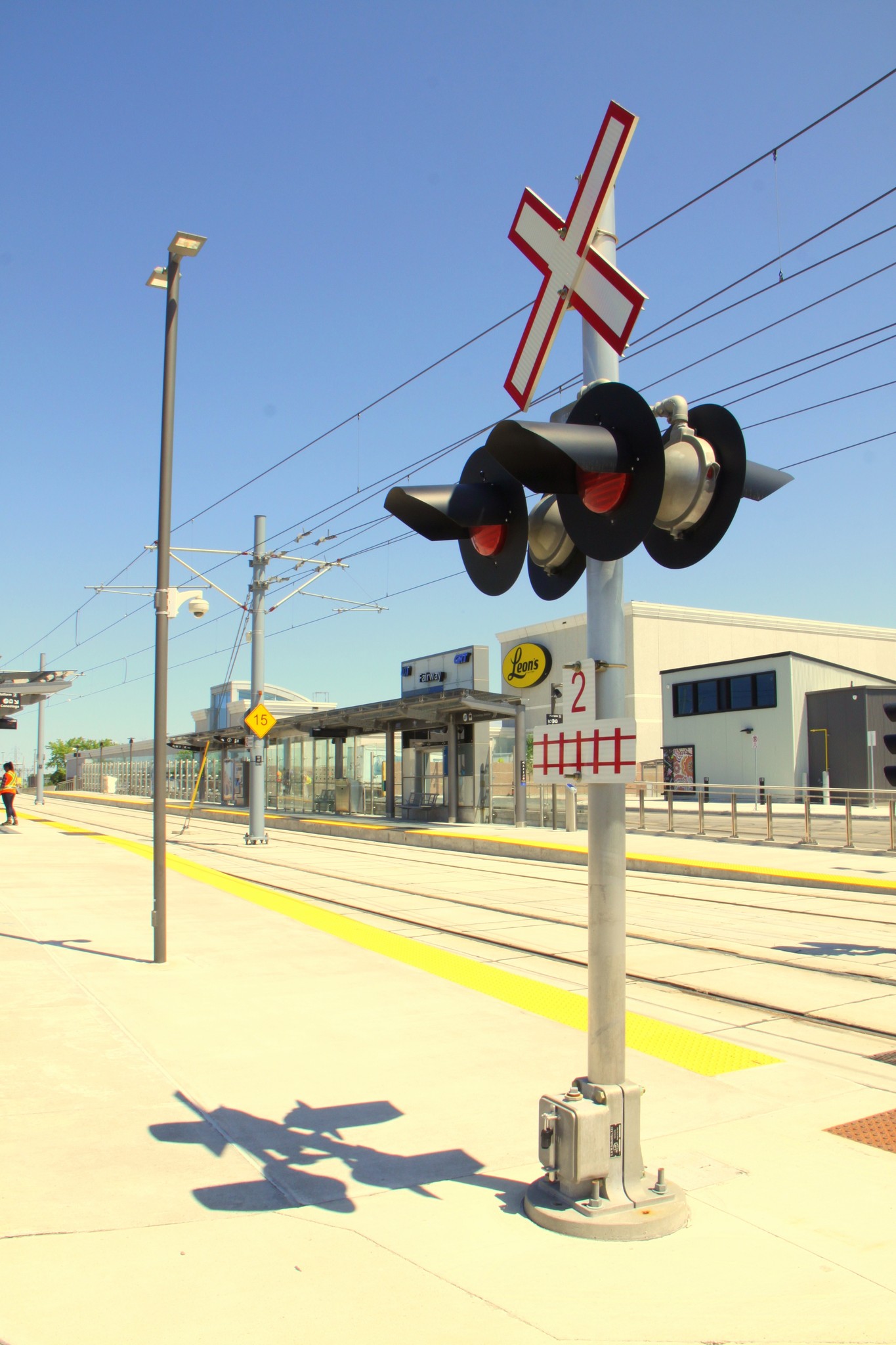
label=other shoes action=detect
[2,820,12,825]
[13,820,18,825]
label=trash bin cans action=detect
[334,778,364,812]
[103,775,117,794]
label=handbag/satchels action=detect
[15,785,21,794]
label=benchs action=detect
[394,792,440,821]
[309,789,335,813]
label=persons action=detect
[0,762,19,826]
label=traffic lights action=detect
[384,384,667,599]
[644,401,796,570]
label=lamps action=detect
[553,689,562,698]
[442,725,448,733]
[458,727,464,734]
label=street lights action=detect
[74,744,82,791]
[146,232,206,966]
[99,741,105,793]
[33,749,37,776]
[1,752,5,780]
[127,736,136,795]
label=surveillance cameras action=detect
[188,599,210,618]
